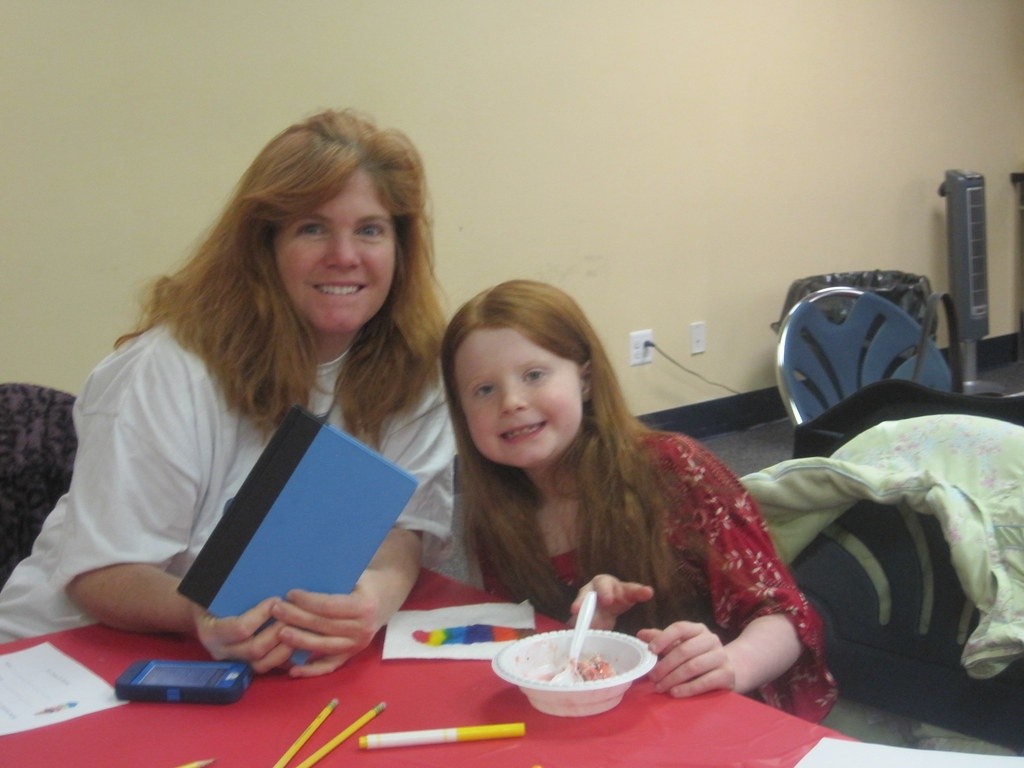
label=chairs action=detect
[773,285,954,432]
[0,382,76,591]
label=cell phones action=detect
[116,660,252,706]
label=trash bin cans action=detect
[793,268,938,411]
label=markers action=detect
[359,722,526,752]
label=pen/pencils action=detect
[175,759,215,768]
[272,698,386,767]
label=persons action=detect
[440,280,838,724]
[0,108,459,676]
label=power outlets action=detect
[629,329,653,365]
[689,321,706,354]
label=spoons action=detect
[549,591,597,686]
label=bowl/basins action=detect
[491,628,658,717]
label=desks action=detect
[0,556,854,768]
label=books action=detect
[177,401,418,664]
